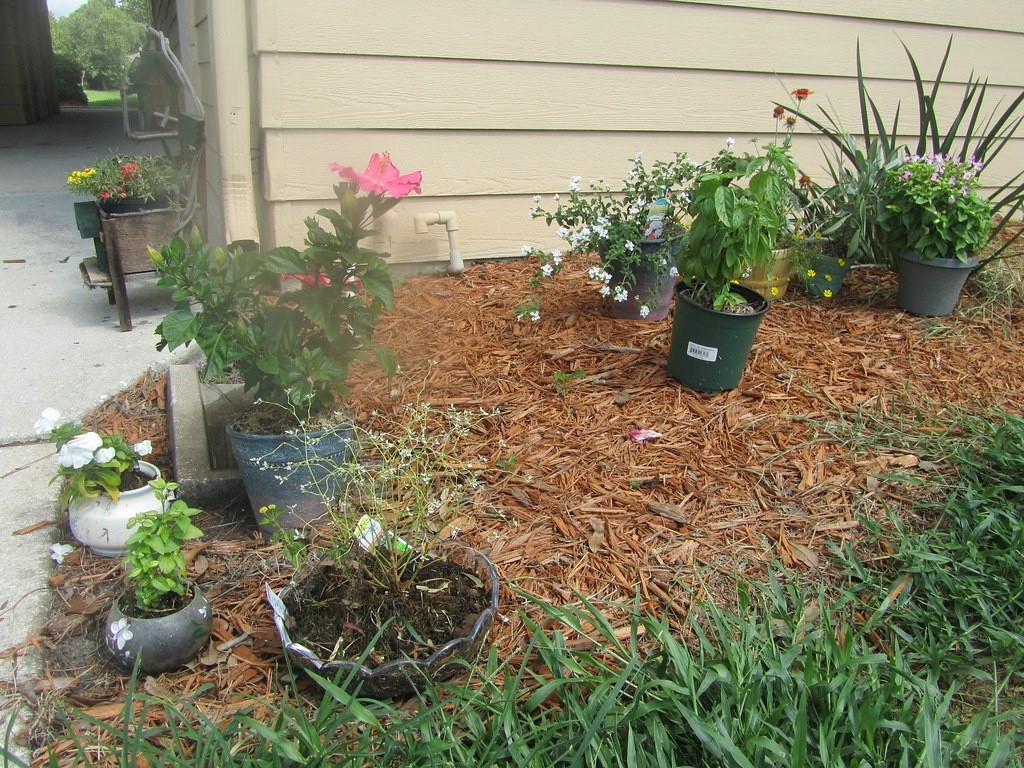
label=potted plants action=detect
[666,144,798,396]
[781,173,869,298]
[104,473,213,675]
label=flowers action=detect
[248,355,529,600]
[146,148,422,424]
[33,406,153,515]
[512,134,735,323]
[66,144,191,203]
[877,151,998,265]
[723,88,817,252]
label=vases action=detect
[597,220,687,323]
[227,403,357,545]
[891,249,982,318]
[67,460,171,558]
[94,188,205,334]
[728,227,794,303]
[271,536,498,704]
